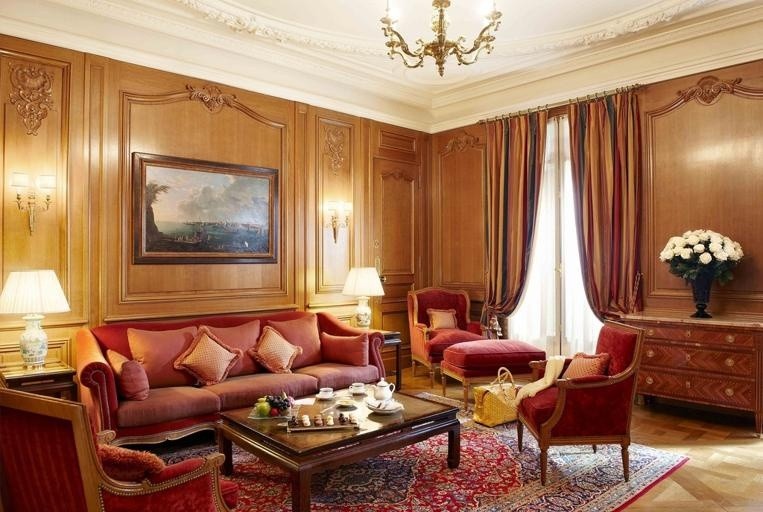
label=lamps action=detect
[342,267,386,330]
[0,270,71,366]
[380,0,503,77]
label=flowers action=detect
[659,228,743,286]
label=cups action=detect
[348,383,364,392]
[319,388,332,397]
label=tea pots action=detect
[370,378,395,400]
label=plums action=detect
[271,408,279,417]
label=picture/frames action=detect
[132,151,279,264]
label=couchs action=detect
[72,312,387,446]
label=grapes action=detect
[266,395,290,411]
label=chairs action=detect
[0,387,239,512]
[515,320,644,487]
[407,286,482,389]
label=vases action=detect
[689,272,713,318]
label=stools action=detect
[440,339,545,410]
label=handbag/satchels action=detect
[472,367,524,427]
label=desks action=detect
[366,329,402,391]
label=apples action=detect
[256,403,270,416]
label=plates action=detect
[336,400,356,407]
[315,394,334,400]
[347,390,366,394]
[367,400,403,415]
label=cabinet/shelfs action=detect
[625,311,763,433]
[0,359,77,400]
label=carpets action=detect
[144,389,690,512]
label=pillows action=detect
[107,349,149,401]
[174,326,242,385]
[320,332,369,367]
[426,309,459,329]
[266,313,321,369]
[198,320,260,377]
[562,353,608,380]
[127,326,198,389]
[247,326,303,374]
[98,444,164,480]
